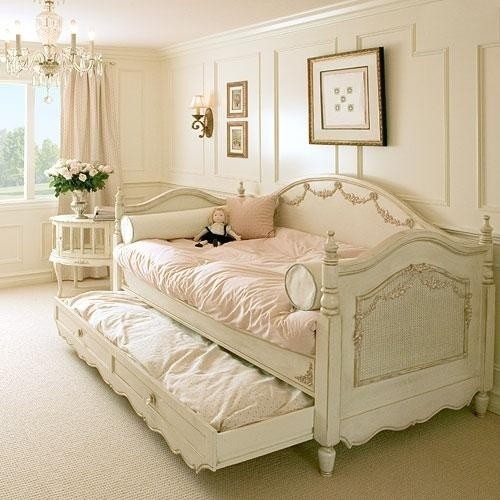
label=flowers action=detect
[43,158,113,198]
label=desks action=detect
[47,213,115,298]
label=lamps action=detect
[188,94,214,138]
[0,0,103,103]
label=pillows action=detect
[226,194,280,239]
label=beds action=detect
[46,173,494,477]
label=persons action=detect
[193,208,243,249]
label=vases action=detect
[69,189,89,220]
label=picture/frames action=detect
[226,121,248,159]
[226,80,248,118]
[306,47,387,147]
